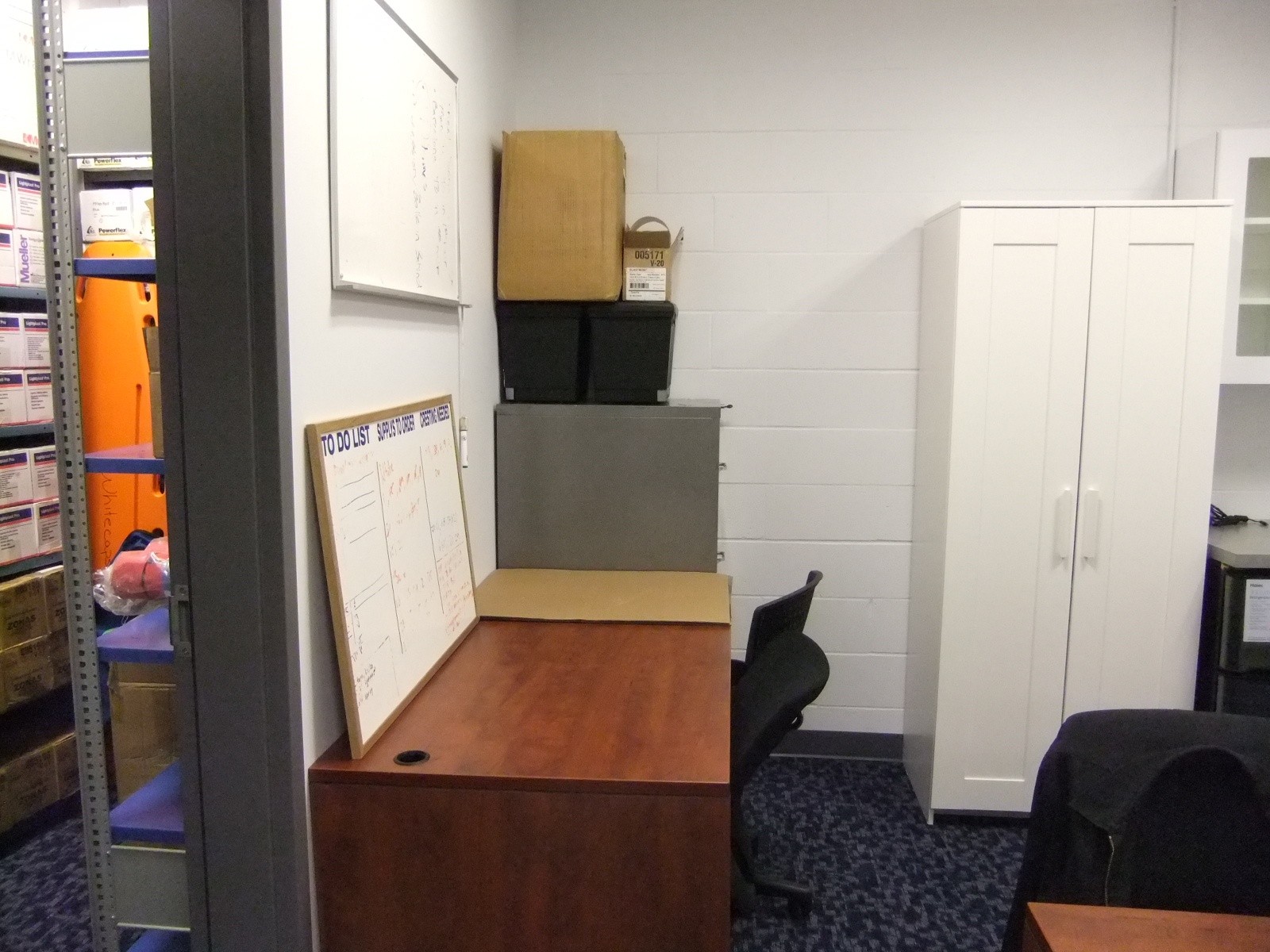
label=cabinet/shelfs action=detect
[497,398,732,573]
[33,0,191,952]
[900,129,1270,828]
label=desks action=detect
[1194,521,1270,712]
[308,621,731,952]
[1021,902,1270,952]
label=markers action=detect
[460,416,468,468]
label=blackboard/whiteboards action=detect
[307,392,480,761]
[326,0,471,308]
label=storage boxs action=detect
[0,156,205,830]
[494,127,683,406]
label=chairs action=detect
[1001,711,1270,952]
[732,570,830,922]
